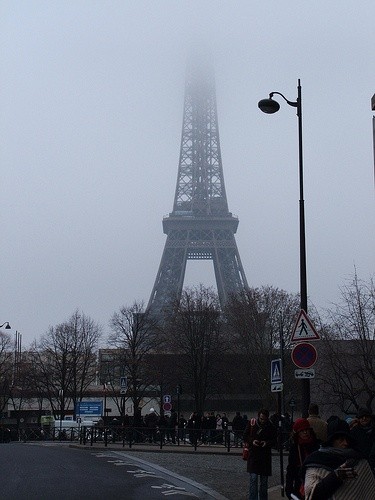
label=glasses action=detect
[360,415,369,418]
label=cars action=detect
[54,420,111,440]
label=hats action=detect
[325,415,352,439]
[293,418,310,432]
[358,406,371,417]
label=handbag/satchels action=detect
[242,441,250,462]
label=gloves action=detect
[334,462,359,481]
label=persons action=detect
[244,409,278,500]
[0,404,375,500]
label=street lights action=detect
[258,76,312,419]
[176,384,180,446]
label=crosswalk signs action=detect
[270,358,282,383]
[290,308,321,343]
